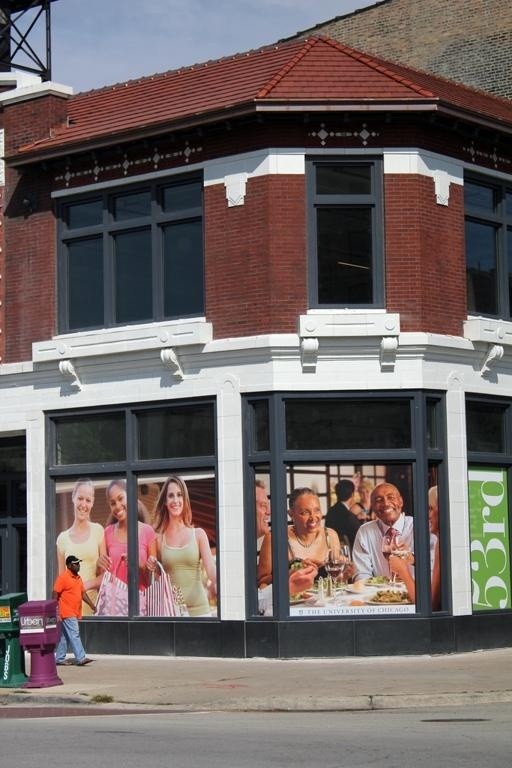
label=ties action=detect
[388,528,399,538]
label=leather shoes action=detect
[77,658,94,666]
[56,659,74,665]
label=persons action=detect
[390,485,441,610]
[354,482,435,583]
[353,479,381,523]
[146,477,218,619]
[258,488,359,594]
[51,556,99,668]
[54,477,104,616]
[255,479,319,615]
[324,471,361,562]
[96,479,157,617]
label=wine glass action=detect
[323,544,351,604]
[381,535,412,585]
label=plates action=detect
[345,583,414,606]
[289,593,319,604]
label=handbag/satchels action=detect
[92,557,190,617]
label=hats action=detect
[66,556,82,564]
